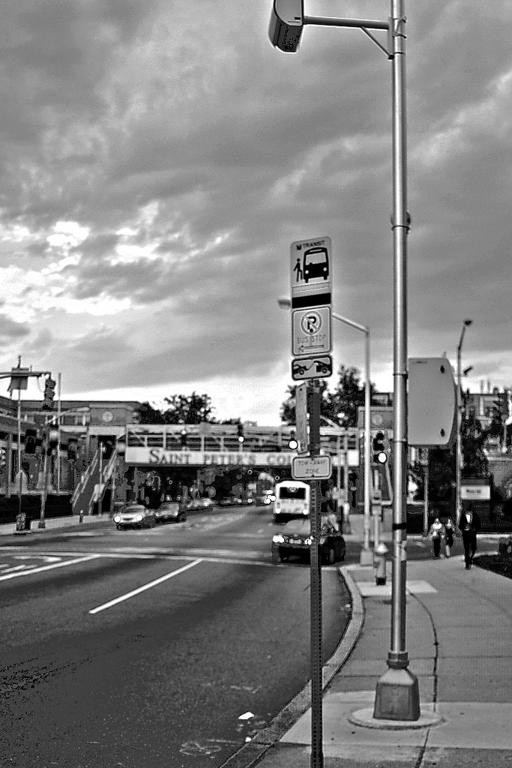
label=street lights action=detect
[279,295,375,564]
[452,317,474,534]
[269,0,419,719]
[38,405,90,530]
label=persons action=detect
[457,508,481,570]
[425,518,446,560]
[444,518,455,560]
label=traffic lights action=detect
[372,428,389,467]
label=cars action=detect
[273,518,348,567]
[115,487,272,529]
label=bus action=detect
[272,480,313,520]
